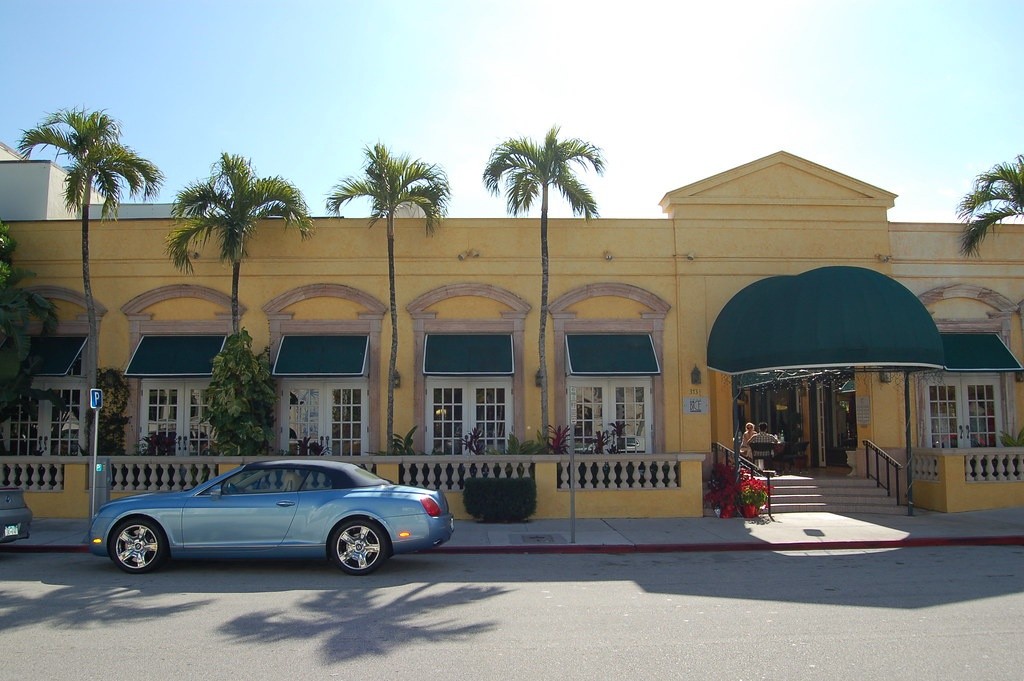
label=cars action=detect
[86,457,455,573]
[0,482,35,550]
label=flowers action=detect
[704,461,775,519]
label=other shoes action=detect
[774,472,782,476]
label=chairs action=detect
[784,441,810,474]
[749,442,773,473]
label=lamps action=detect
[458,249,480,261]
[687,251,696,260]
[606,255,614,263]
[691,363,702,384]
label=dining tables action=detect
[774,441,794,447]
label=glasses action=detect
[747,427,753,429]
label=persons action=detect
[740,422,758,463]
[747,422,778,456]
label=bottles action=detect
[780,430,784,442]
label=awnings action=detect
[272,335,371,378]
[705,265,944,374]
[123,335,227,377]
[564,335,662,377]
[939,332,1024,373]
[22,335,89,376]
[422,333,515,376]
